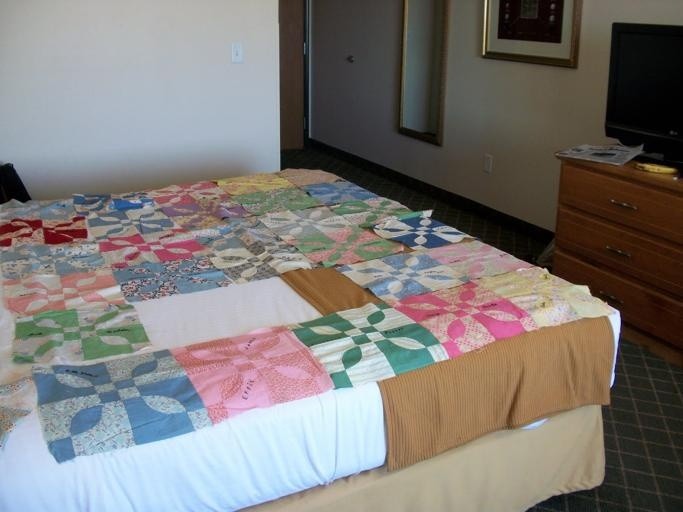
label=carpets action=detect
[528,338,681,512]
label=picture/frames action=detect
[481,0,583,70]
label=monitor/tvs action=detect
[604,21,683,171]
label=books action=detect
[554,143,645,166]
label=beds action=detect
[0,167,621,511]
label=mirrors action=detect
[397,1,451,147]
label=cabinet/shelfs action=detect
[552,141,682,353]
